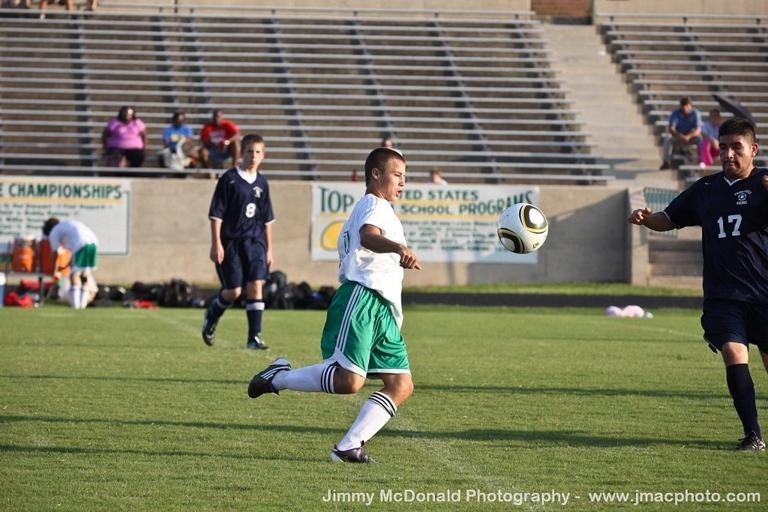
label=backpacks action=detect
[125,270,333,308]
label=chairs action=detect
[0,244,53,307]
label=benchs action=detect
[0,0,768,184]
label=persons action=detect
[659,97,724,171]
[102,105,242,179]
[201,134,274,351]
[430,167,448,187]
[627,117,768,454]
[248,138,420,463]
[43,217,99,310]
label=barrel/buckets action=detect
[11,235,35,273]
[38,239,58,275]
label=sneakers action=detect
[247,357,291,398]
[247,336,269,350]
[330,439,374,463]
[735,431,765,450]
[202,311,216,347]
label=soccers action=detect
[498,203,548,253]
